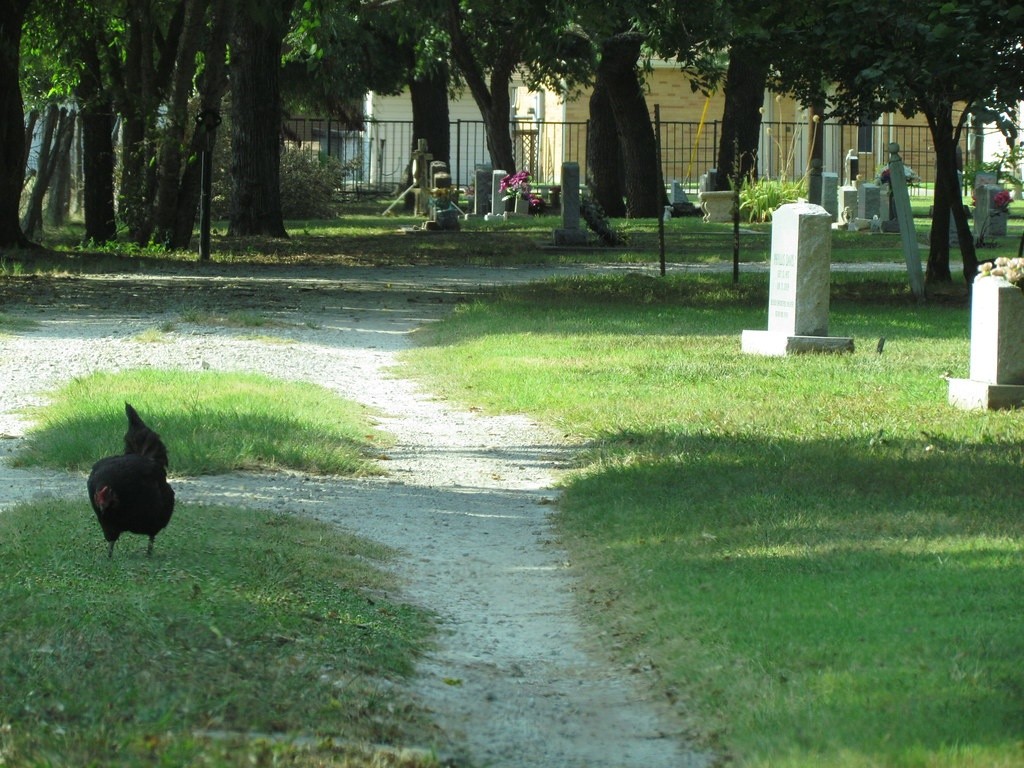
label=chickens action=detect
[87,401,175,558]
